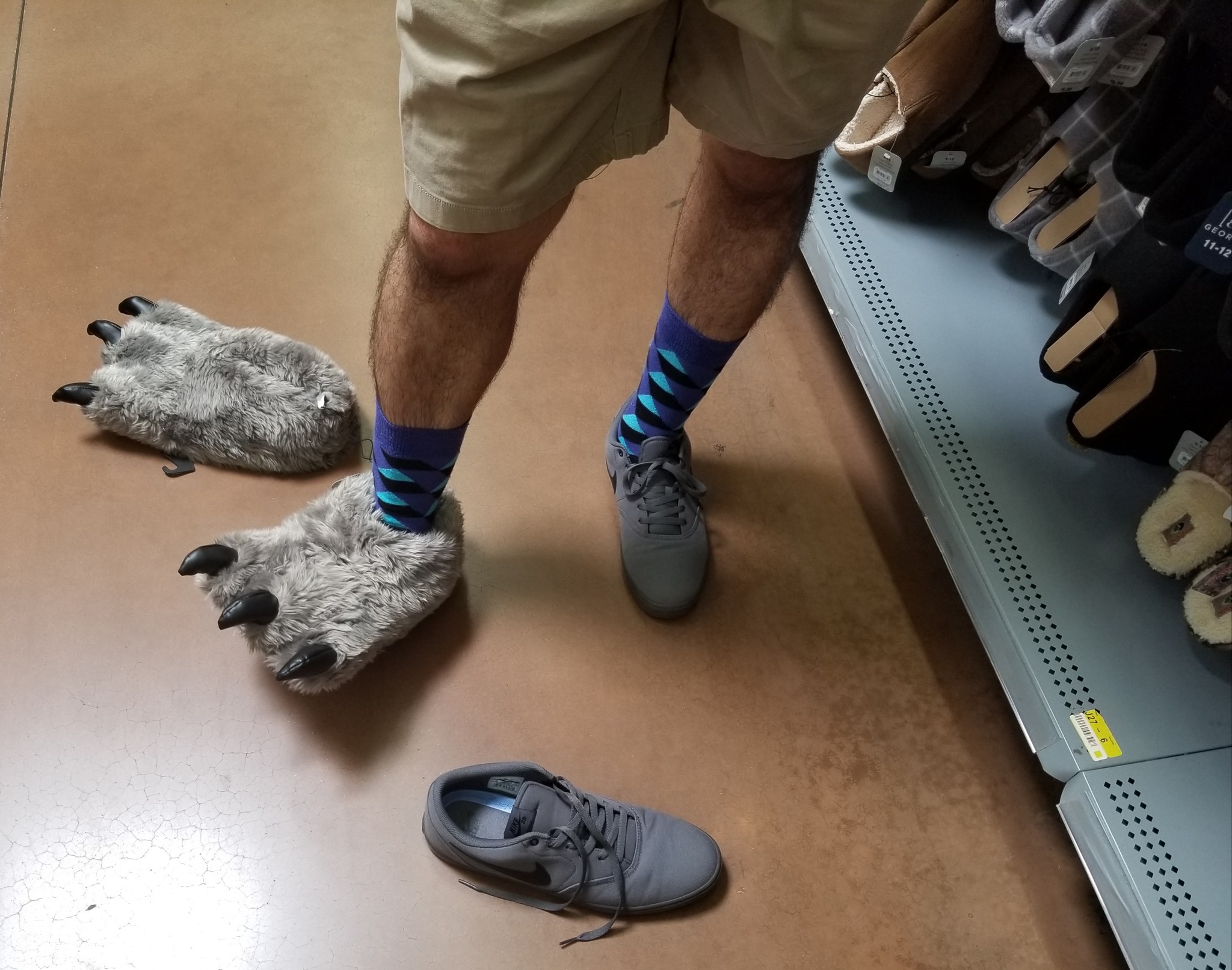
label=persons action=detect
[180,0,931,700]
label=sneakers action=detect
[606,390,709,620]
[422,762,721,910]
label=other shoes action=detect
[835,1,1232,649]
[52,296,361,472]
[178,471,464,694]
